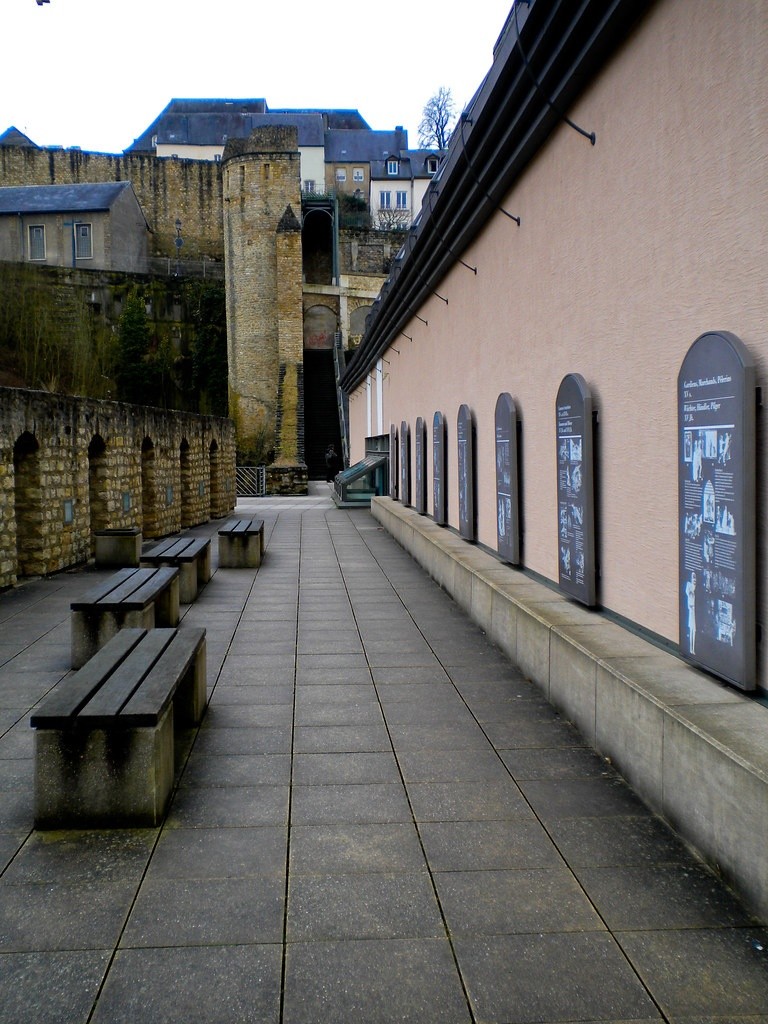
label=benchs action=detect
[218,518,265,569]
[141,536,213,605]
[28,624,211,825]
[69,566,182,668]
